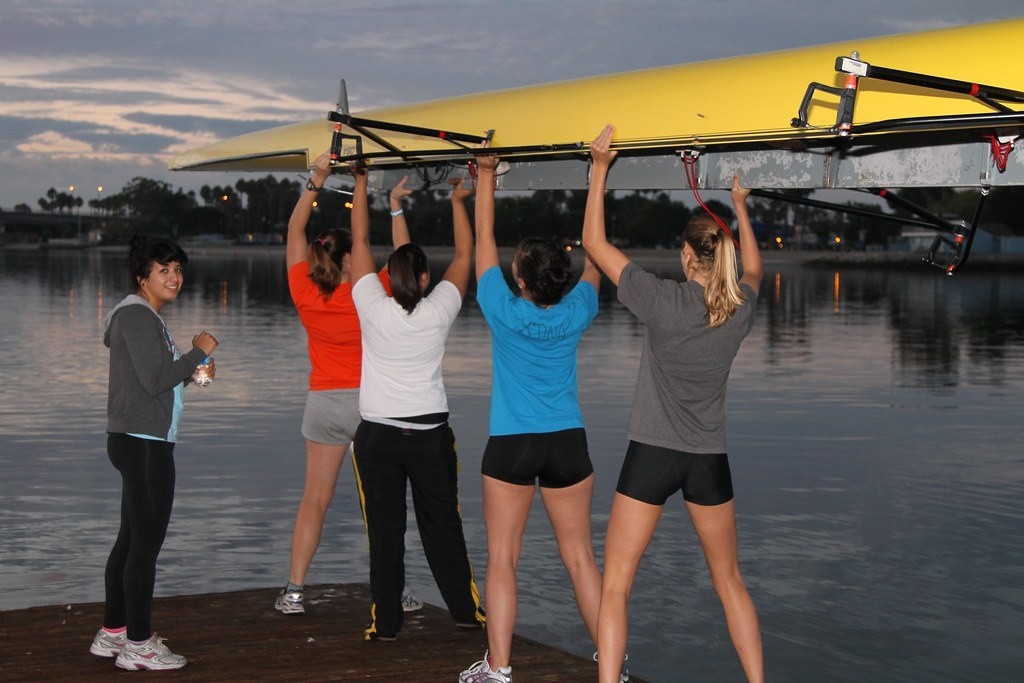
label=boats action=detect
[167,19,1024,278]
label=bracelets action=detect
[390,209,403,217]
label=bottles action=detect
[193,351,213,389]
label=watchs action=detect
[307,179,323,192]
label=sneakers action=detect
[618,662,630,683]
[90,628,188,670]
[458,649,512,683]
[401,586,424,611]
[274,584,305,614]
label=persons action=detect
[88,237,220,672]
[582,123,767,683]
[458,152,630,683]
[349,160,487,641]
[275,146,424,614]
